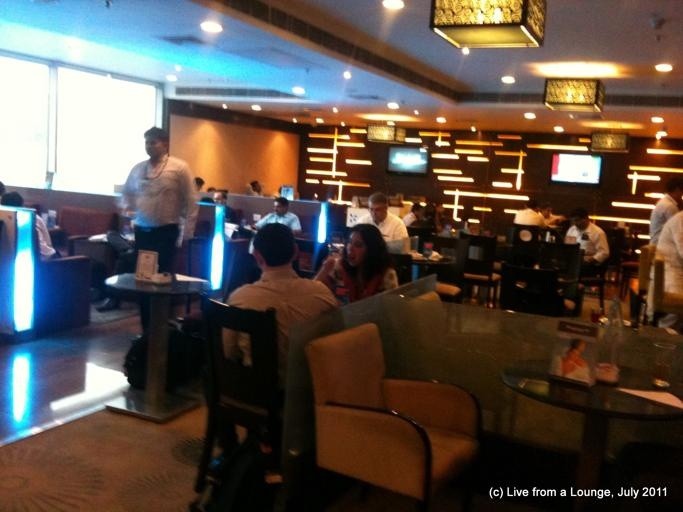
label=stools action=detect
[618,261,641,300]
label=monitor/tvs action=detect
[386,145,431,178]
[548,152,602,186]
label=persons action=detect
[538,202,566,241]
[245,180,267,198]
[194,176,205,192]
[0,191,61,264]
[435,202,445,231]
[513,199,546,242]
[212,192,238,223]
[121,129,197,332]
[207,224,340,478]
[403,203,424,228]
[253,197,302,237]
[313,224,400,306]
[562,207,610,292]
[649,174,683,239]
[95,218,185,312]
[552,338,592,382]
[646,211,683,335]
[356,192,409,243]
[411,194,436,224]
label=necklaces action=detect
[143,155,169,180]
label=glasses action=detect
[366,206,385,213]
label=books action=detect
[548,320,601,388]
[136,250,158,282]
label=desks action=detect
[221,237,252,303]
[65,233,108,256]
[103,272,211,423]
[414,252,456,280]
[502,241,581,318]
[501,358,683,512]
[46,226,62,234]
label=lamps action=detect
[590,133,631,154]
[543,78,605,113]
[430,0,548,49]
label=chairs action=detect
[432,235,465,305]
[346,206,369,228]
[389,252,413,286]
[648,252,682,328]
[407,225,432,253]
[303,322,486,512]
[637,244,654,320]
[511,222,540,244]
[575,263,606,317]
[194,292,281,497]
[460,230,501,308]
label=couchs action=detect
[285,273,683,462]
[176,199,224,298]
[1,204,91,339]
[225,192,327,274]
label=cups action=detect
[651,342,676,389]
